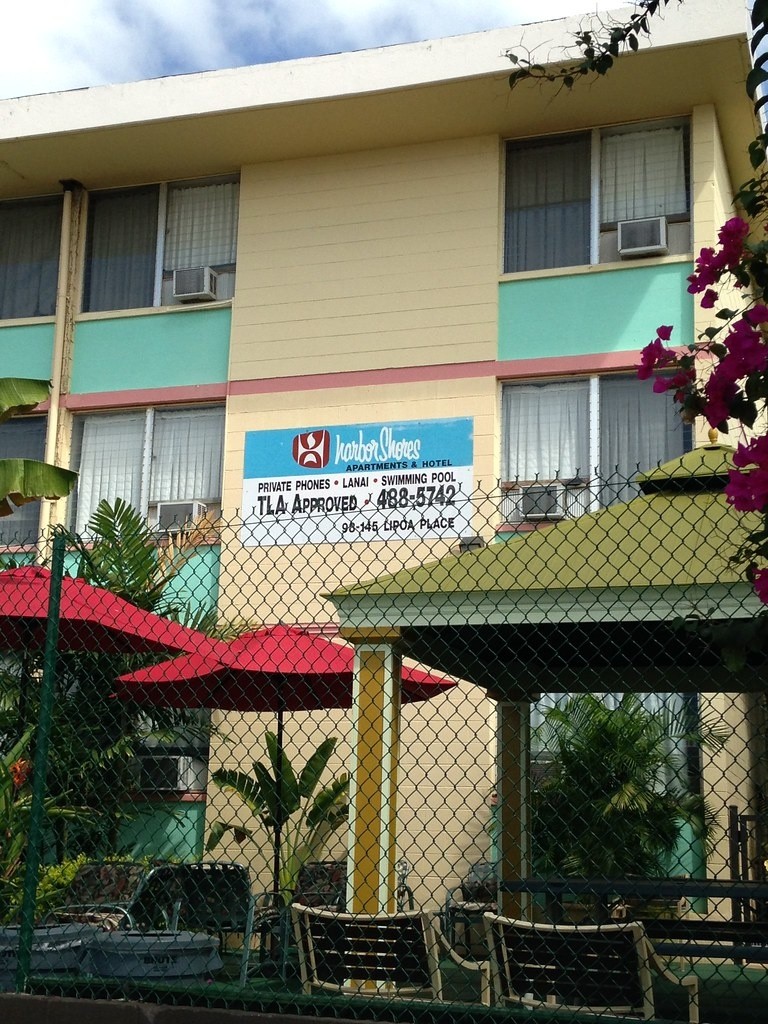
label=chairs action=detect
[482,913,700,1024]
[166,861,277,976]
[289,904,493,1008]
[396,884,444,961]
[444,887,492,961]
[41,856,164,932]
[260,860,347,980]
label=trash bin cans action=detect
[87,928,223,977]
[0,920,95,971]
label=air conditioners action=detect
[519,485,568,519]
[137,754,194,792]
[156,501,208,533]
[618,216,671,259]
[172,266,218,301]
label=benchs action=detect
[462,877,768,961]
